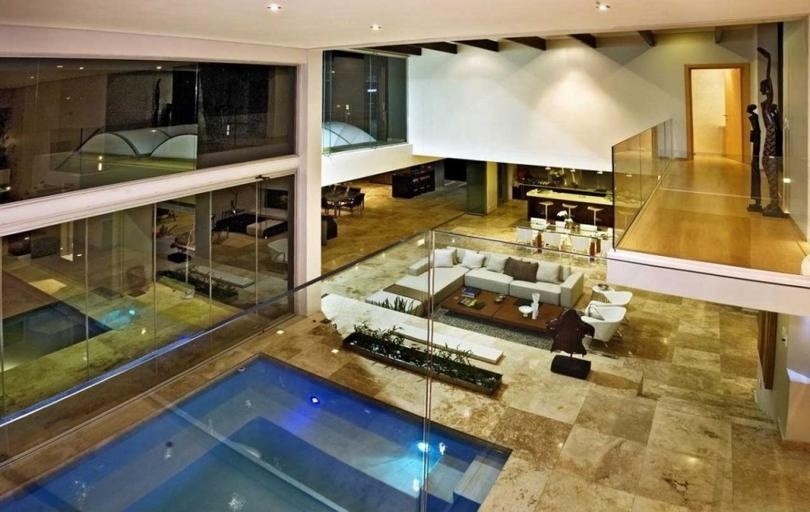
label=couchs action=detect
[365,244,586,318]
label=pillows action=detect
[431,245,561,285]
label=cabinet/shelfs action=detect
[391,168,435,199]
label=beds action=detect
[217,213,289,240]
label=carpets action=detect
[429,303,561,354]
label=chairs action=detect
[265,238,287,273]
[580,281,634,350]
[544,310,595,380]
[321,185,365,218]
[516,218,601,262]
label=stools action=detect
[538,201,604,233]
